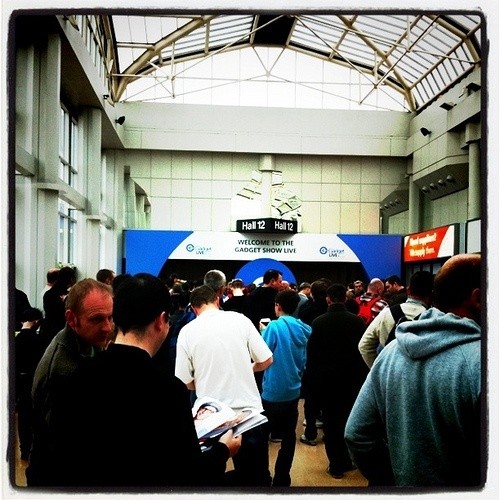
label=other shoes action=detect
[300,435,316,445]
[302,418,323,427]
[271,438,282,441]
[327,467,343,479]
[343,465,357,472]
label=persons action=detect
[25,273,243,488]
[32,278,113,408]
[175,285,273,487]
[193,402,222,425]
[13,266,115,462]
[198,410,252,442]
[159,270,408,446]
[307,284,370,479]
[243,269,282,395]
[259,290,312,486]
[344,253,487,488]
[358,270,436,369]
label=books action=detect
[191,395,268,453]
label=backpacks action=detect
[355,296,381,326]
[385,305,421,346]
